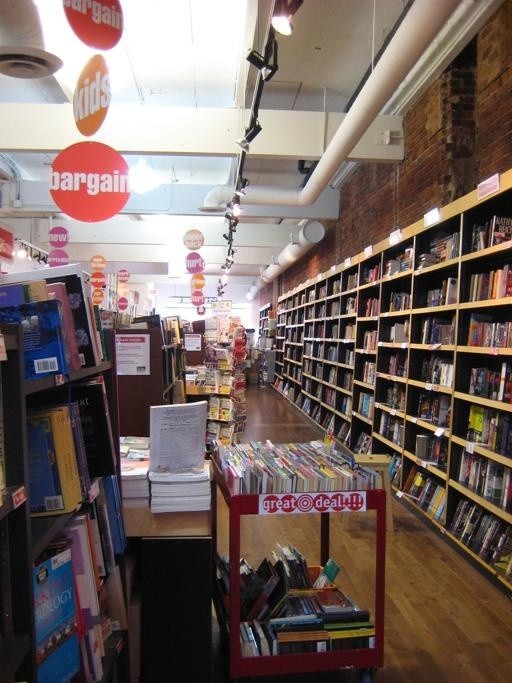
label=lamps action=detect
[233,0,305,197]
[0,0,63,81]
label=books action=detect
[2,263,376,682]
[258,215,511,581]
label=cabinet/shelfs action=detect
[400,211,463,533]
[447,185,512,594]
[207,449,387,680]
[0,316,138,683]
[258,304,275,385]
[371,234,418,493]
[115,316,189,438]
[274,252,384,456]
